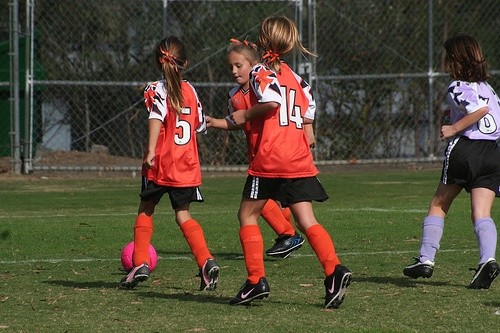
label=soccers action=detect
[121,241,158,274]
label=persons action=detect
[204,38,307,259]
[229,16,353,311]
[402,34,500,290]
[120,35,220,292]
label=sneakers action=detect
[469,258,500,290]
[230,277,270,306]
[266,231,305,256]
[120,263,150,289]
[199,258,220,292]
[403,256,435,279]
[323,264,352,309]
